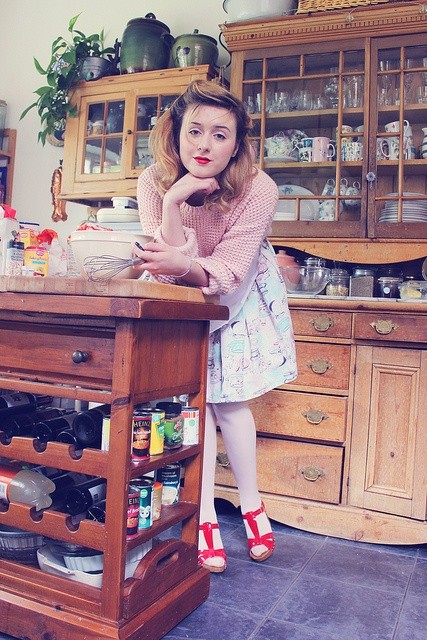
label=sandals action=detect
[197,522,227,573]
[241,501,275,561]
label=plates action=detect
[263,156,295,163]
[378,192,426,222]
[277,184,320,221]
[319,200,342,221]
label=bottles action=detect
[0,389,110,448]
[22,461,106,523]
[0,465,56,511]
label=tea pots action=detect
[263,131,298,156]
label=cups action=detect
[344,181,361,206]
[332,178,347,196]
[243,55,426,114]
[243,119,426,163]
[321,178,335,195]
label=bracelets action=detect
[170,257,193,280]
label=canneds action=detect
[130,412,151,461]
[180,406,199,445]
[126,485,140,541]
[129,479,153,528]
[24,246,48,278]
[156,463,180,506]
[135,408,165,455]
[153,481,162,521]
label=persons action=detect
[129,77,298,572]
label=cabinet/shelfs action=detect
[2,287,231,640]
[215,0,427,264]
[54,64,230,214]
[207,295,426,546]
[0,127,16,211]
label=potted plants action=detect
[18,11,116,146]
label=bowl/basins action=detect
[0,527,46,565]
[277,265,330,295]
[41,537,98,557]
[70,230,155,279]
[222,1,297,22]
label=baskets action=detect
[296,0,384,15]
